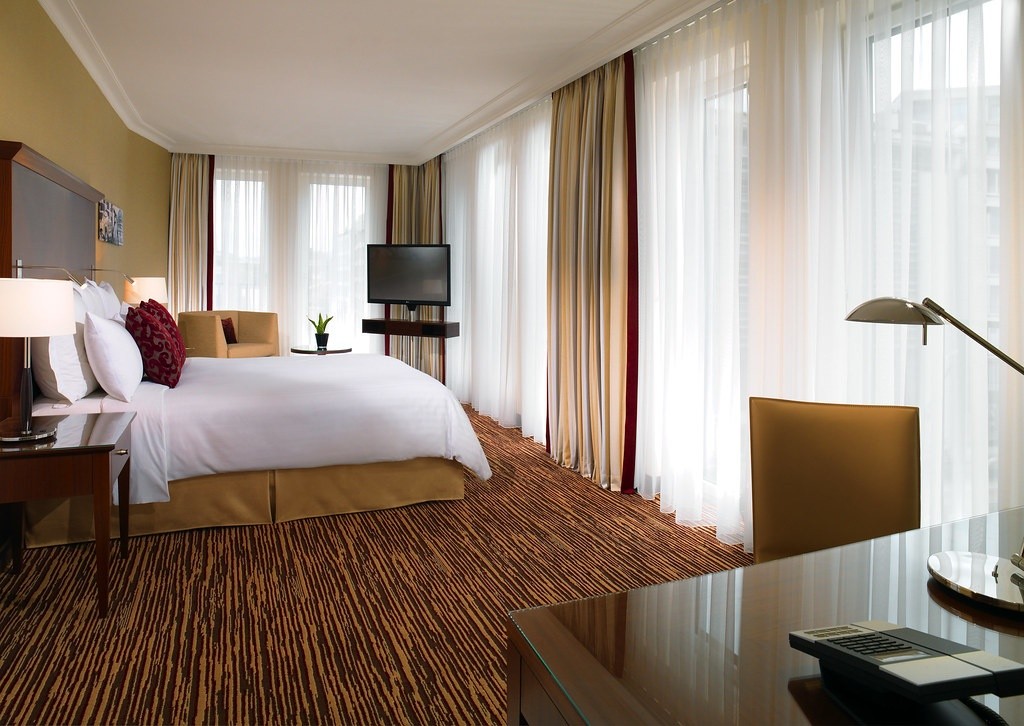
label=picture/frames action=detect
[97,199,124,247]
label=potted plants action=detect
[309,312,334,349]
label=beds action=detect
[0,140,493,550]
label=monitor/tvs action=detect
[367,244,451,306]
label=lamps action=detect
[16,260,85,289]
[125,277,168,303]
[0,278,77,445]
[843,297,1024,614]
[91,264,135,284]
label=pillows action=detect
[30,276,185,402]
[221,317,238,344]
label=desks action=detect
[291,345,353,355]
[506,508,1023,726]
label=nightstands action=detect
[0,412,136,620]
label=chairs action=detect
[749,395,921,564]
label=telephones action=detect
[785,617,1024,726]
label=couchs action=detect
[178,311,280,358]
